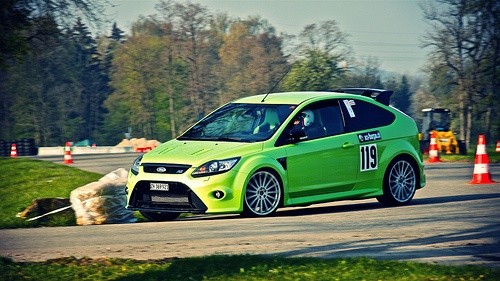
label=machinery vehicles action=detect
[417,106,467,156]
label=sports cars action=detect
[124,87,426,224]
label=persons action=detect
[289,109,324,139]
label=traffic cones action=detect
[466,135,497,185]
[63,142,74,164]
[91,143,96,147]
[9,143,18,158]
[427,132,444,162]
[495,139,500,152]
